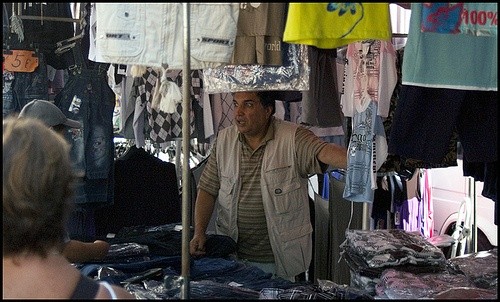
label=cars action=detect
[426,143,498,260]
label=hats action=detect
[15,99,81,129]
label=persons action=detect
[189,90,347,282]
[3,98,136,299]
